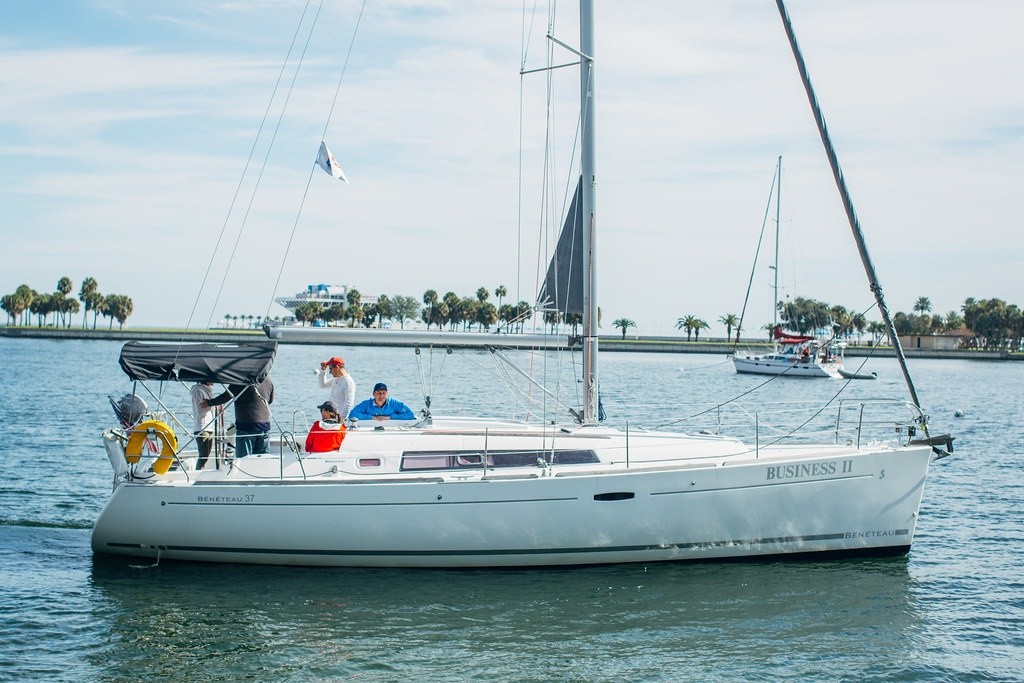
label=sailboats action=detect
[726,154,852,380]
[88,1,958,569]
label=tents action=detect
[118,339,294,481]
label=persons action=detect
[821,350,841,364]
[348,382,414,421]
[189,378,275,470]
[800,351,811,364]
[305,357,356,453]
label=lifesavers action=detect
[125,420,178,476]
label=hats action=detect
[317,401,338,412]
[323,358,345,367]
[374,383,387,392]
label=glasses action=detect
[375,391,387,395]
[320,409,328,414]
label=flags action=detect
[314,141,350,185]
